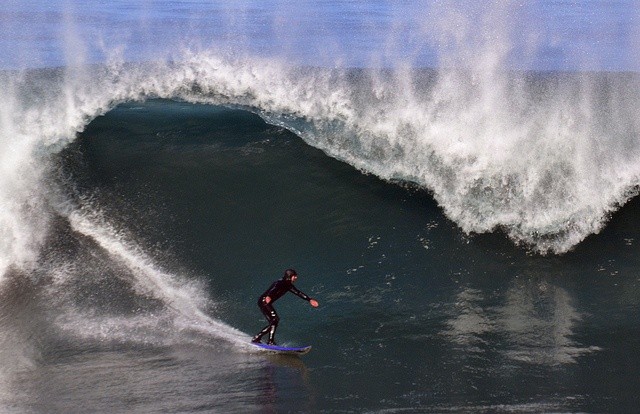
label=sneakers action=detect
[252,335,262,344]
[267,340,277,345]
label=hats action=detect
[281,268,296,278]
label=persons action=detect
[252,269,319,345]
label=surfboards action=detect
[247,342,312,355]
